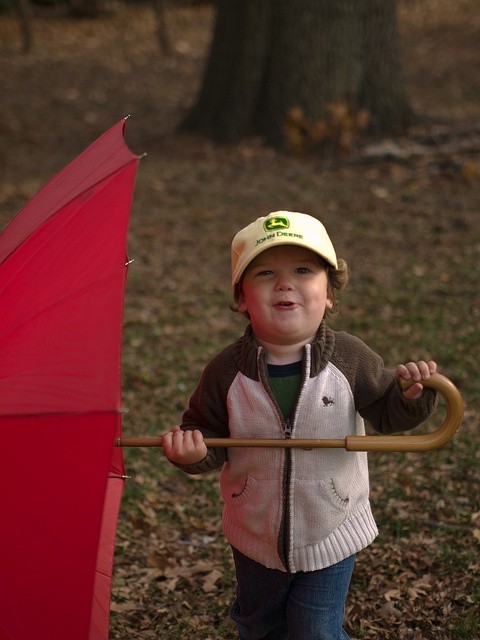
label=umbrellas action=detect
[0,112,465,640]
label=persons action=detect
[160,209,437,640]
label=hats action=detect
[230,210,338,301]
